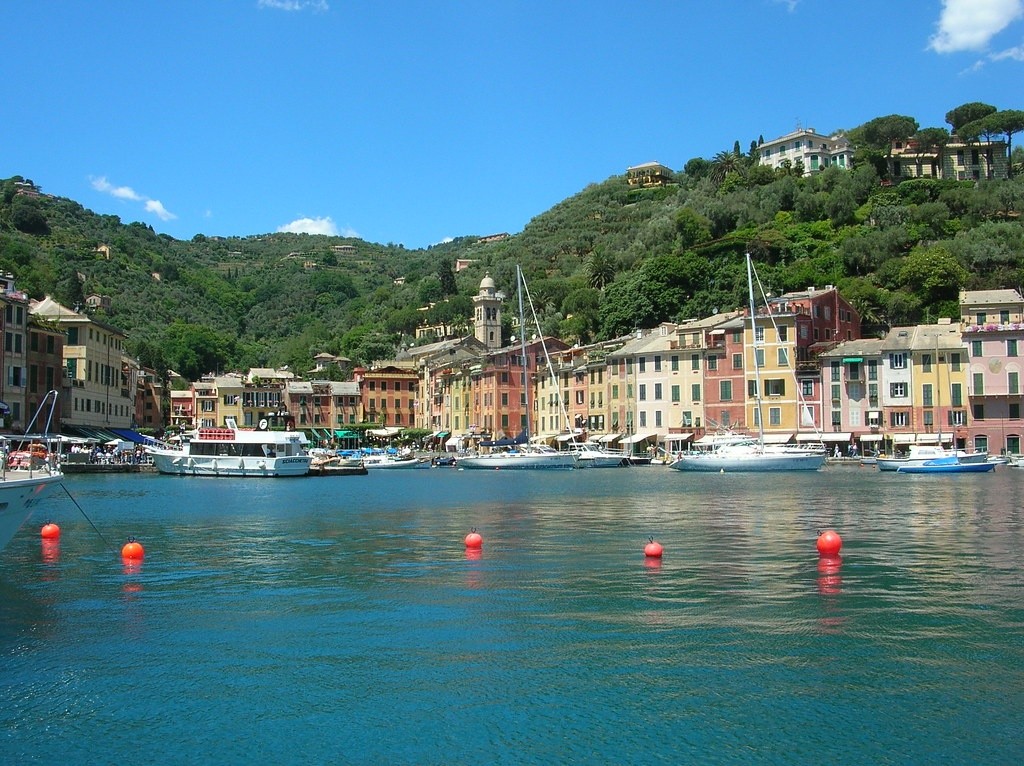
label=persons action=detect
[5,444,141,465]
[833,442,879,458]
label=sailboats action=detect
[668,252,829,472]
[454,264,583,471]
[875,333,990,471]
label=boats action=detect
[897,456,997,474]
[986,454,1024,467]
[307,442,628,476]
[860,457,877,464]
[142,418,315,476]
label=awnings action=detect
[893,433,953,444]
[64,424,156,445]
[530,433,745,446]
[335,427,460,446]
[758,433,883,443]
